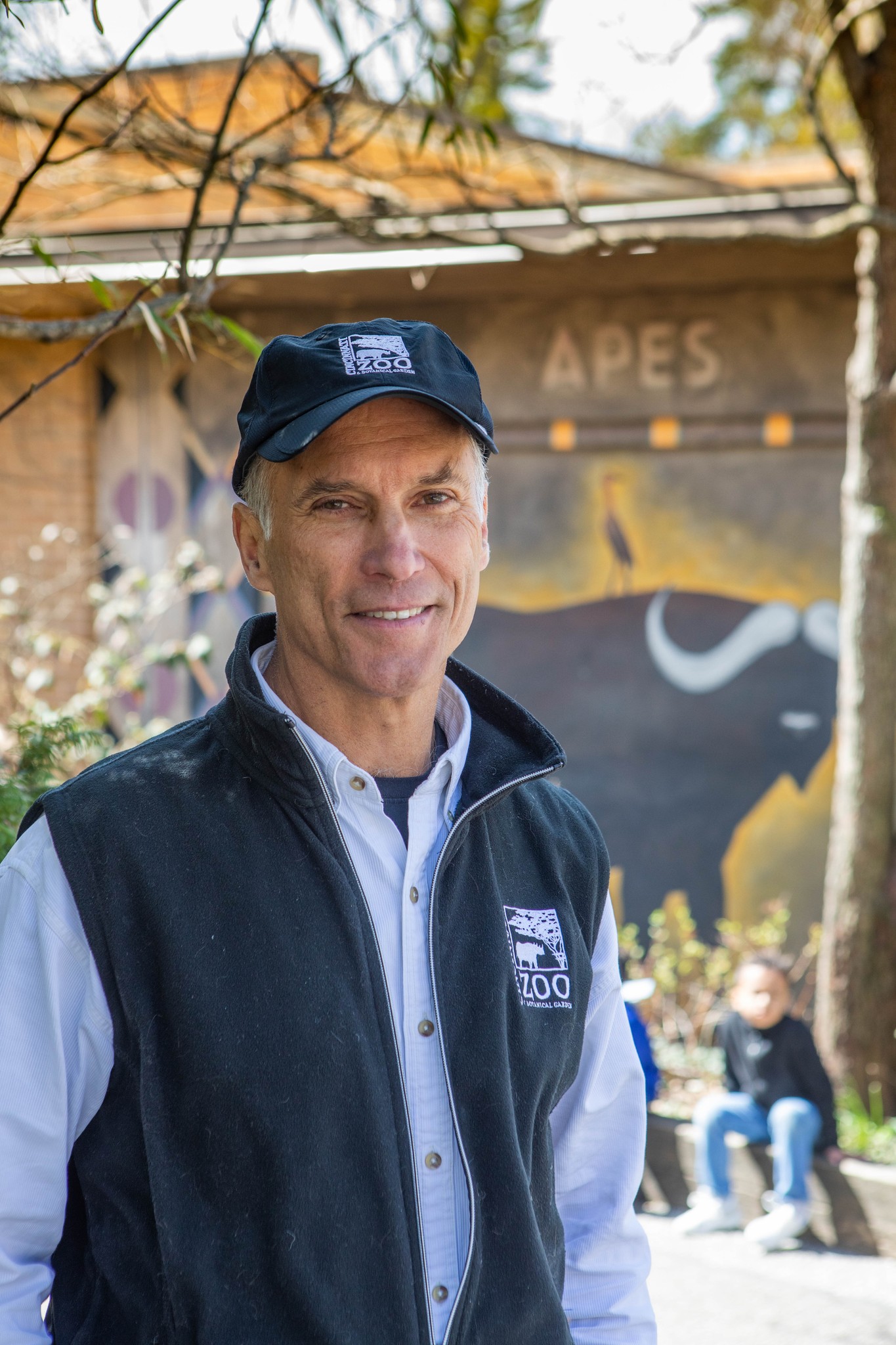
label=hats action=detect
[232,321,498,500]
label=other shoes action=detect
[672,1185,739,1235]
[745,1201,810,1253]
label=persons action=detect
[676,962,842,1251]
[0,317,657,1345]
[623,1001,661,1103]
